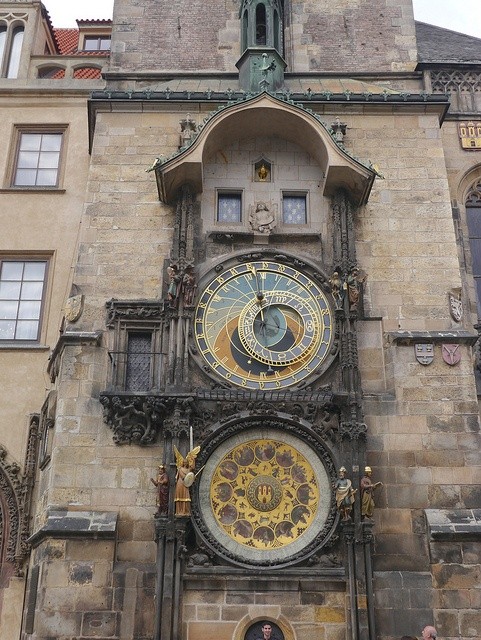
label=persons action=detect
[328,268,345,311]
[346,266,360,314]
[250,623,281,640]
[183,266,197,308]
[417,626,439,640]
[359,462,379,517]
[174,458,193,517]
[332,463,355,523]
[248,197,275,236]
[150,464,170,517]
[165,264,181,308]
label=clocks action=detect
[193,417,343,568]
[189,253,338,392]
